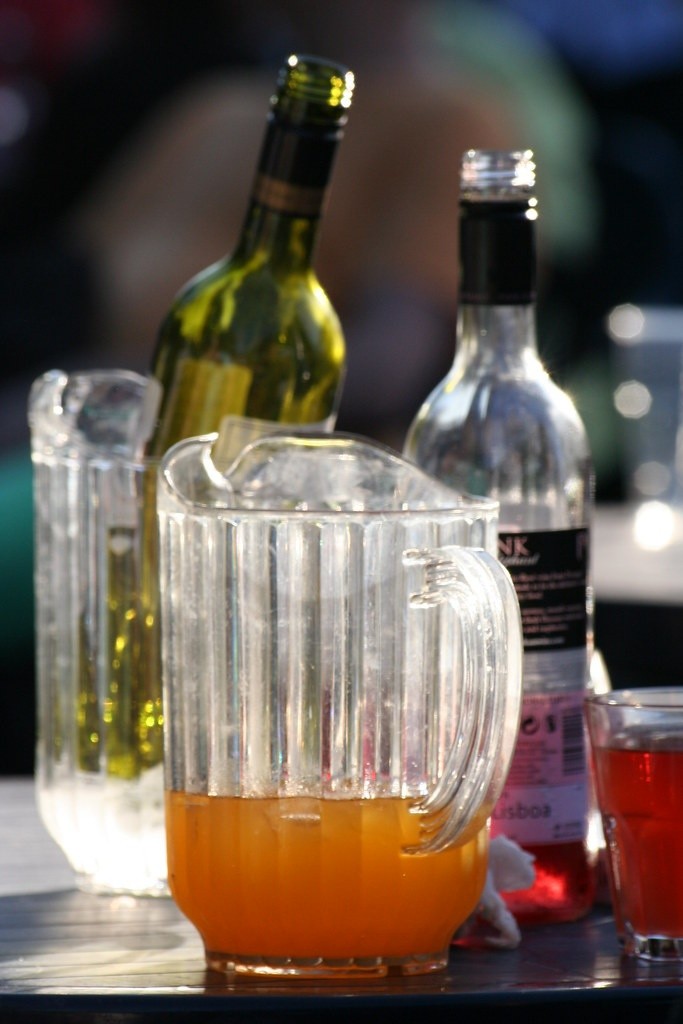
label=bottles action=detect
[404,142,605,930]
[76,53,363,790]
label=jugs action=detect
[152,424,526,984]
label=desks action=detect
[0,776,683,1024]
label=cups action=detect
[24,369,348,896]
[581,686,682,965]
